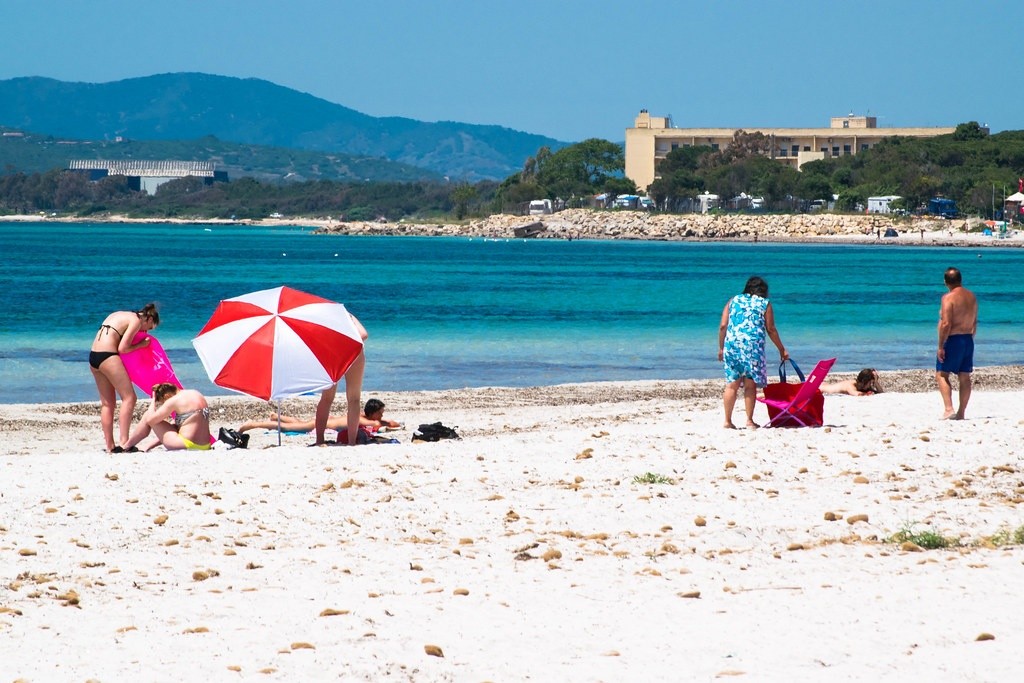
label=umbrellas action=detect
[984,219,996,231]
[189,285,364,447]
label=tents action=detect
[884,229,898,237]
[1004,191,1024,202]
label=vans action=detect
[809,200,827,212]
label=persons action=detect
[718,276,789,429]
[755,368,883,396]
[316,312,369,446]
[110,382,212,453]
[935,267,977,421]
[89,303,159,454]
[237,398,401,434]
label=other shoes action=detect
[111,446,144,453]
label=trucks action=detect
[529,199,554,215]
[867,194,905,215]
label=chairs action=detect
[756,357,837,428]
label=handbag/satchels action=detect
[764,357,825,427]
[219,427,250,449]
